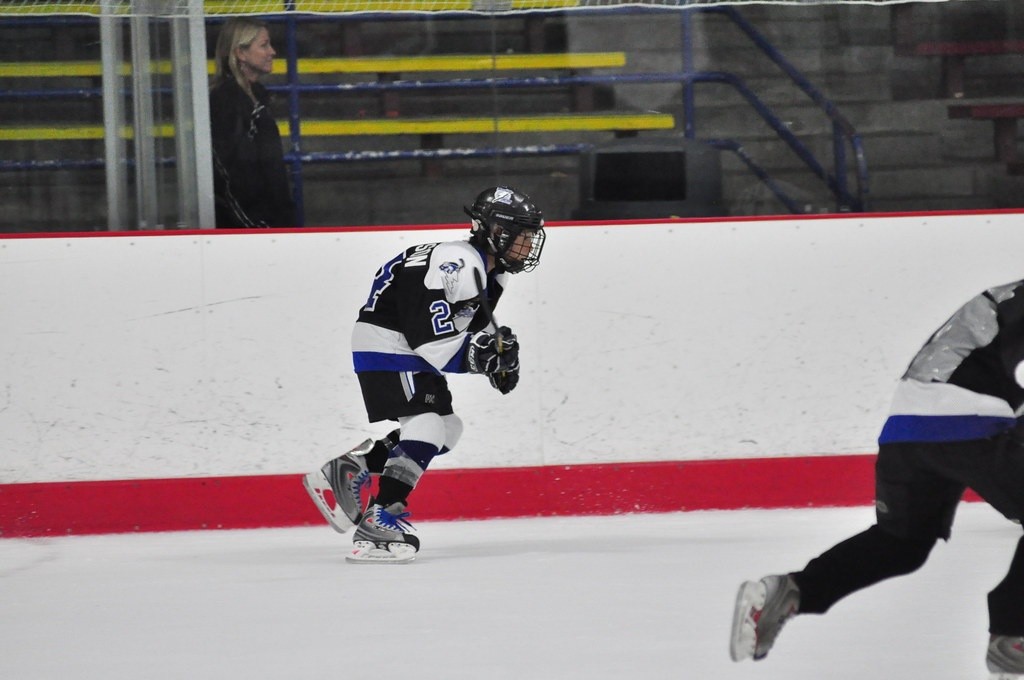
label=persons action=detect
[303,185,546,565]
[210,16,298,229]
[731,278,1024,680]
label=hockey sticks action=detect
[473,266,506,377]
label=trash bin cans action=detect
[577,135,726,220]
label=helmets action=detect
[462,187,544,274]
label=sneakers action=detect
[986,633,1024,680]
[731,574,801,662]
[303,438,376,534]
[345,493,419,563]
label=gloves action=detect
[463,325,521,394]
